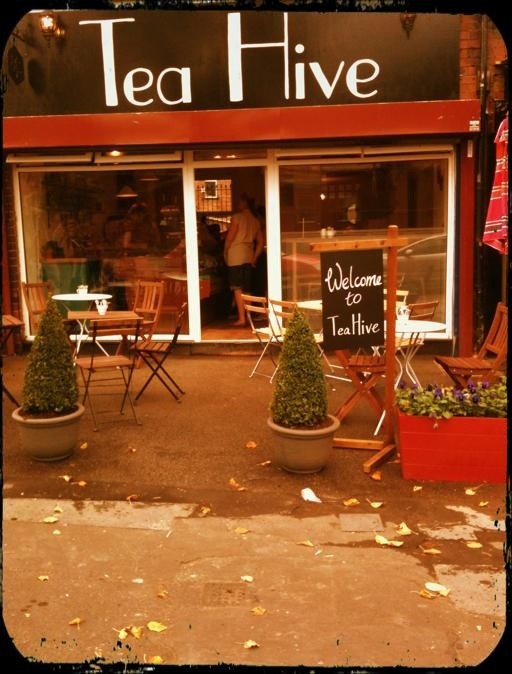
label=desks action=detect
[68,311,138,396]
[54,293,112,366]
[0,313,26,408]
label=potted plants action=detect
[266,306,341,474]
[12,291,86,462]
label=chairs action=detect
[16,281,74,358]
[73,317,144,431]
[128,302,185,401]
[236,285,508,426]
[116,279,168,368]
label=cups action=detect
[96,300,108,316]
[77,284,89,299]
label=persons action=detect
[250,208,266,316]
[198,214,208,230]
[113,203,150,308]
[223,193,265,327]
[200,224,222,255]
